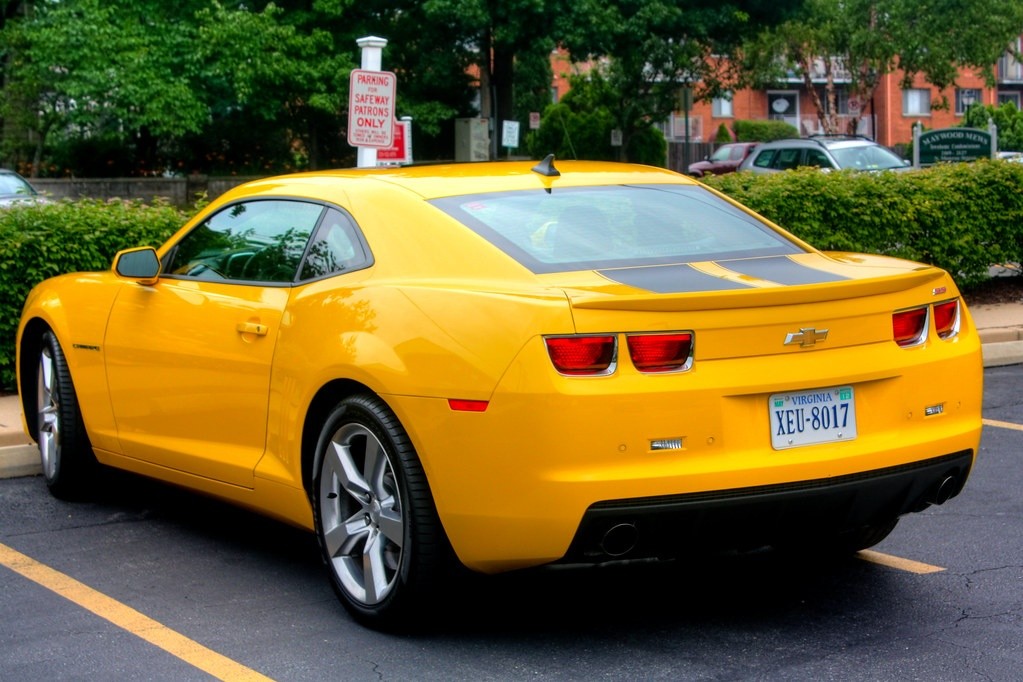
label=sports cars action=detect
[16,153,985,627]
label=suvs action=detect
[688,141,762,178]
[737,134,917,171]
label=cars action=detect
[0,169,52,204]
